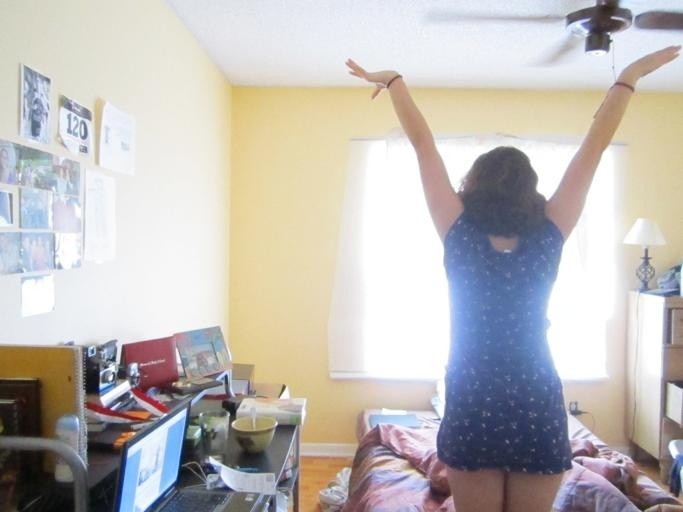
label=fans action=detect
[429,0,683,91]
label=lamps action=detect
[583,29,610,58]
[621,217,670,292]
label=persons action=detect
[345,46,683,512]
[0,78,81,274]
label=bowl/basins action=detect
[231,417,279,453]
[235,452,271,470]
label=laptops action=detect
[111,396,263,512]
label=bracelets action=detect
[386,74,402,89]
[615,82,635,92]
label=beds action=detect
[345,407,682,512]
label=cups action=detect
[55,414,86,484]
[208,455,228,467]
[199,409,230,453]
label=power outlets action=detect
[569,401,578,416]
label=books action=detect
[0,376,45,478]
[235,396,308,425]
[83,379,137,432]
[0,397,23,486]
[166,375,223,395]
[0,343,87,469]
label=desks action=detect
[0,372,306,512]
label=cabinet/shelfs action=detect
[625,289,682,483]
